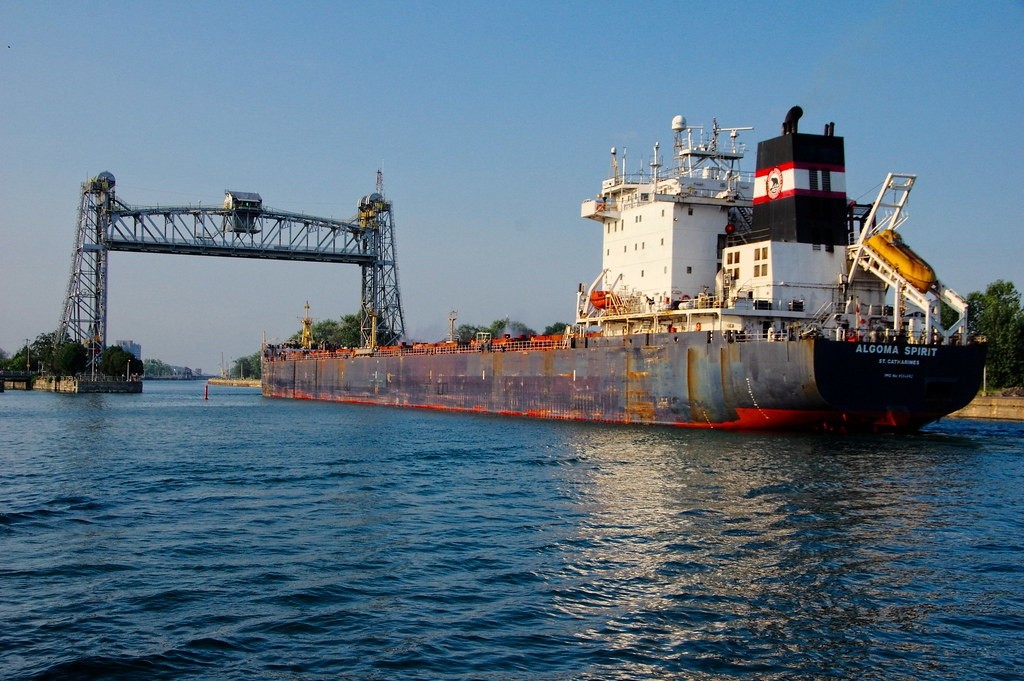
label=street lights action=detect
[127,360,131,381]
[92,319,102,381]
[26,339,30,373]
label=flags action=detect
[856,304,867,324]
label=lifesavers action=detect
[681,295,690,300]
[846,328,857,342]
[696,321,701,331]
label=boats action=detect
[677,299,701,309]
[869,230,937,294]
[260,104,990,432]
[590,292,621,309]
